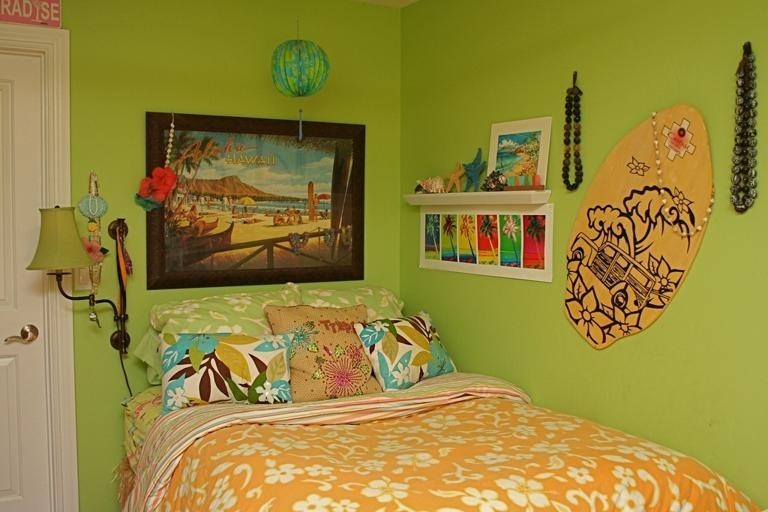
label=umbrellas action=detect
[237,197,256,206]
[317,193,331,200]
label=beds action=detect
[119,384,760,512]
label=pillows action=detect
[149,282,405,335]
[265,304,383,403]
[158,332,295,414]
[354,310,457,393]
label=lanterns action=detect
[271,40,330,100]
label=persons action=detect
[192,194,248,218]
[187,204,207,237]
[273,205,303,225]
[244,218,265,224]
[321,209,330,220]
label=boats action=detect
[183,220,234,266]
[180,217,219,234]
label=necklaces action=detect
[651,110,716,238]
[730,42,757,213]
[561,70,583,191]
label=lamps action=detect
[22,206,132,355]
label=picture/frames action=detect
[142,109,368,291]
